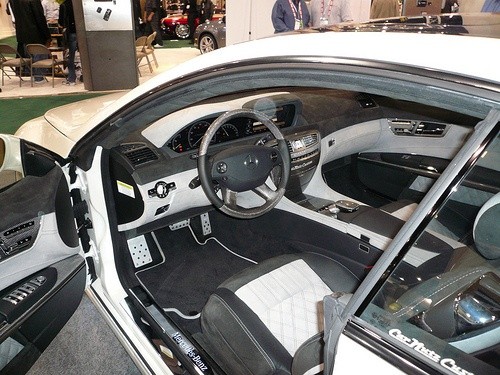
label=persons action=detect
[271,0,310,34]
[133,0,164,48]
[307,0,354,28]
[373,15,470,36]
[57,0,84,86]
[182,0,215,45]
[293,22,355,34]
[369,0,459,20]
[7,0,69,84]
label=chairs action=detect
[0,31,159,88]
[200,192,500,375]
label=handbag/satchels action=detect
[160,7,167,18]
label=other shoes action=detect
[62,81,76,86]
[34,78,44,84]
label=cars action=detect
[193,14,226,55]
[161,14,226,40]
[0,13,500,374]
